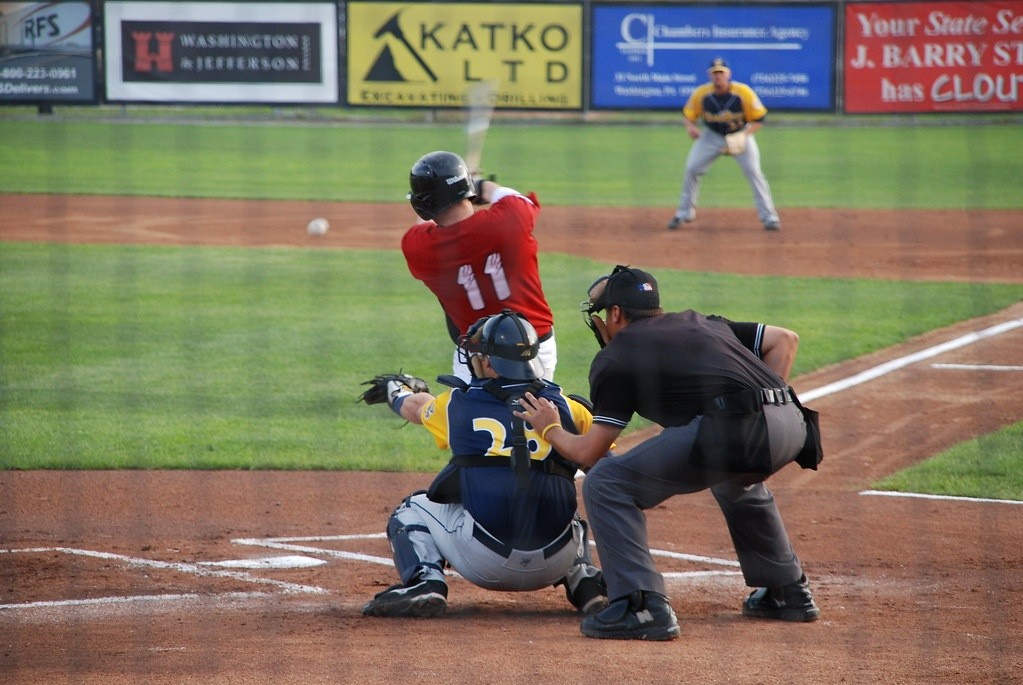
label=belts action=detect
[762,388,793,403]
[472,526,573,558]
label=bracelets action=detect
[542,423,561,441]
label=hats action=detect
[708,58,730,74]
[592,268,659,310]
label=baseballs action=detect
[308,217,330,237]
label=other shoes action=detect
[765,222,778,230]
[671,216,683,227]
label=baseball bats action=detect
[466,77,500,170]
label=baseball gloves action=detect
[355,366,430,430]
[719,130,747,155]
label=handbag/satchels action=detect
[788,386,823,471]
[689,408,773,476]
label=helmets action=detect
[409,150,479,220]
[484,314,546,380]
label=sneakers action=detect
[361,580,448,617]
[580,595,680,640]
[742,575,820,620]
[574,578,609,616]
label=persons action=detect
[512,266,820,639]
[401,151,558,380]
[359,309,610,616]
[668,58,780,232]
